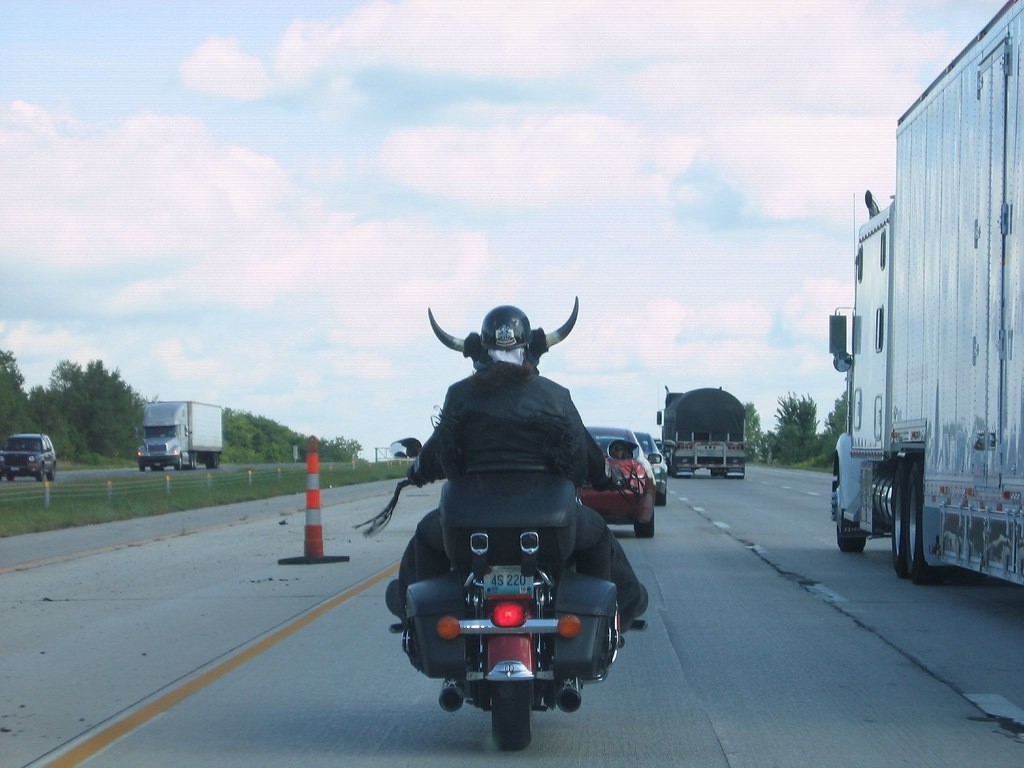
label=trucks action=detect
[830,2,1024,588]
[657,383,747,479]
[137,399,223,470]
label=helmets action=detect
[481,305,534,352]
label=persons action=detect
[386,297,648,633]
[611,443,626,458]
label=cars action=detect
[631,431,671,506]
[575,426,662,540]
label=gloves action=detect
[405,465,423,488]
[595,466,631,492]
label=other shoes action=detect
[385,576,410,628]
[616,583,654,634]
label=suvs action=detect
[0,433,57,482]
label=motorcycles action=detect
[388,433,648,752]
